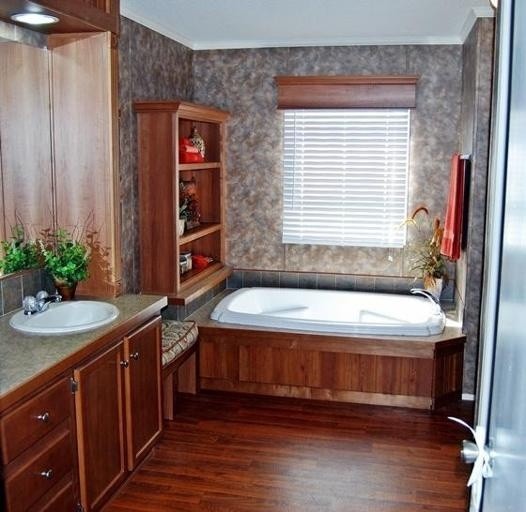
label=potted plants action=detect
[399,205,451,302]
[29,199,102,301]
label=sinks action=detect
[7,299,120,335]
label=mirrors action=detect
[0,34,57,282]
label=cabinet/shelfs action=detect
[133,97,233,304]
[0,318,167,512]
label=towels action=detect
[440,151,466,262]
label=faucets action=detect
[410,287,442,315]
[22,289,63,314]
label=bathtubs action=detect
[209,288,445,337]
